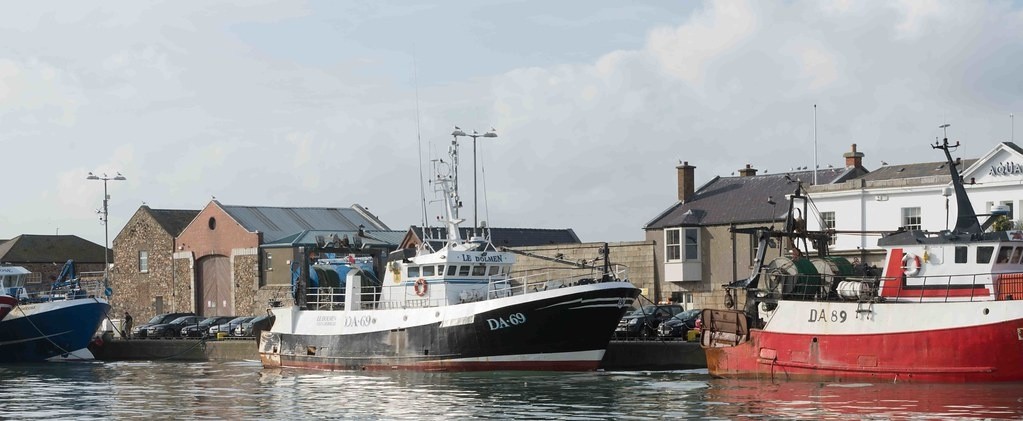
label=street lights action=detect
[1010,114,1013,143]
[938,124,950,138]
[451,128,498,239]
[86,171,128,333]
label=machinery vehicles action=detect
[33,259,89,304]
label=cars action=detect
[210,316,255,339]
[248,315,276,339]
[656,306,704,343]
[235,317,256,338]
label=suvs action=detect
[180,315,242,340]
[616,303,685,342]
[132,311,197,340]
[146,316,207,340]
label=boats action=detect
[0,259,113,366]
[0,262,33,304]
[701,123,1023,390]
[259,125,642,376]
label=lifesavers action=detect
[414,278,428,297]
[900,253,921,277]
